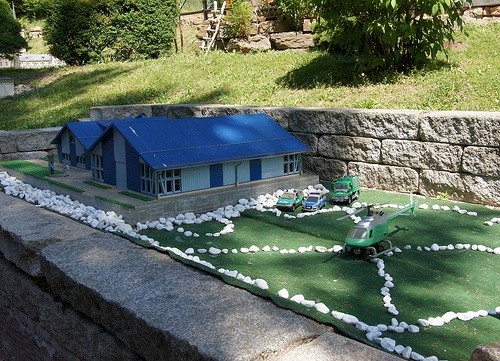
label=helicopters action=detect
[332,187,419,260]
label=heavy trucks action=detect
[330,175,361,203]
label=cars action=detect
[277,188,305,211]
[302,191,327,212]
